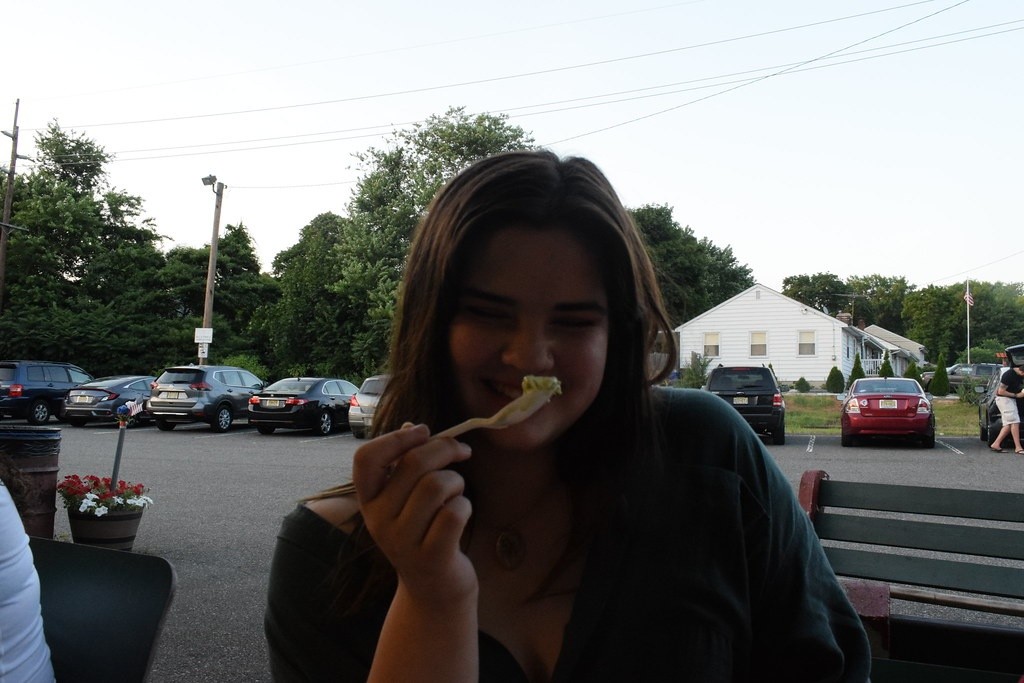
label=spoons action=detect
[389,384,561,467]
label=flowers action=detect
[56,475,151,517]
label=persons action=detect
[0,448,60,683]
[990,366,1024,454]
[265,150,872,683]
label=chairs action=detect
[719,377,732,387]
[30,536,178,683]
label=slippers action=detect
[1015,449,1024,455]
[991,448,1009,453]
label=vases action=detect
[66,508,143,552]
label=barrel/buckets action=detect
[0,425,62,539]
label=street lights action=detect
[196,175,224,365]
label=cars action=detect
[247,377,360,437]
[974,344,1024,448]
[837,376,935,447]
[59,374,158,427]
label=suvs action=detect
[704,365,785,444]
[0,360,95,426]
[145,363,269,432]
[920,363,1003,393]
[347,375,390,439]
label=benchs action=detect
[797,469,1024,683]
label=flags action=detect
[964,291,974,306]
